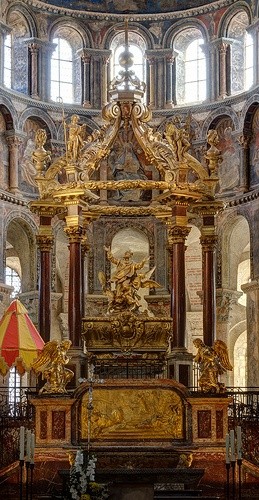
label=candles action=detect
[237,426,241,458]
[19,426,24,460]
[230,430,235,461]
[225,434,229,462]
[31,434,34,465]
[27,430,30,462]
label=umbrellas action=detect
[0,298,45,415]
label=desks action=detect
[58,468,206,489]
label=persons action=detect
[164,122,190,158]
[63,115,81,156]
[32,129,51,163]
[104,245,148,291]
[193,338,233,393]
[34,340,74,391]
